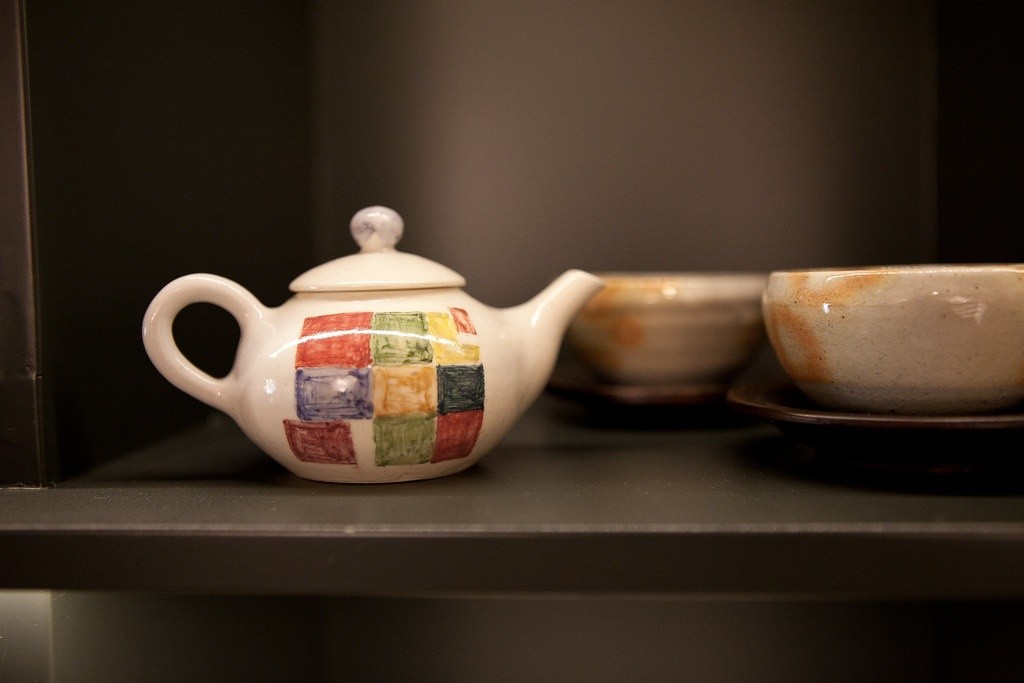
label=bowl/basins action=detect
[566,270,770,383]
[760,263,1024,411]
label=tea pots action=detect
[143,207,607,485]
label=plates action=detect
[724,380,1024,434]
[559,378,726,400]
